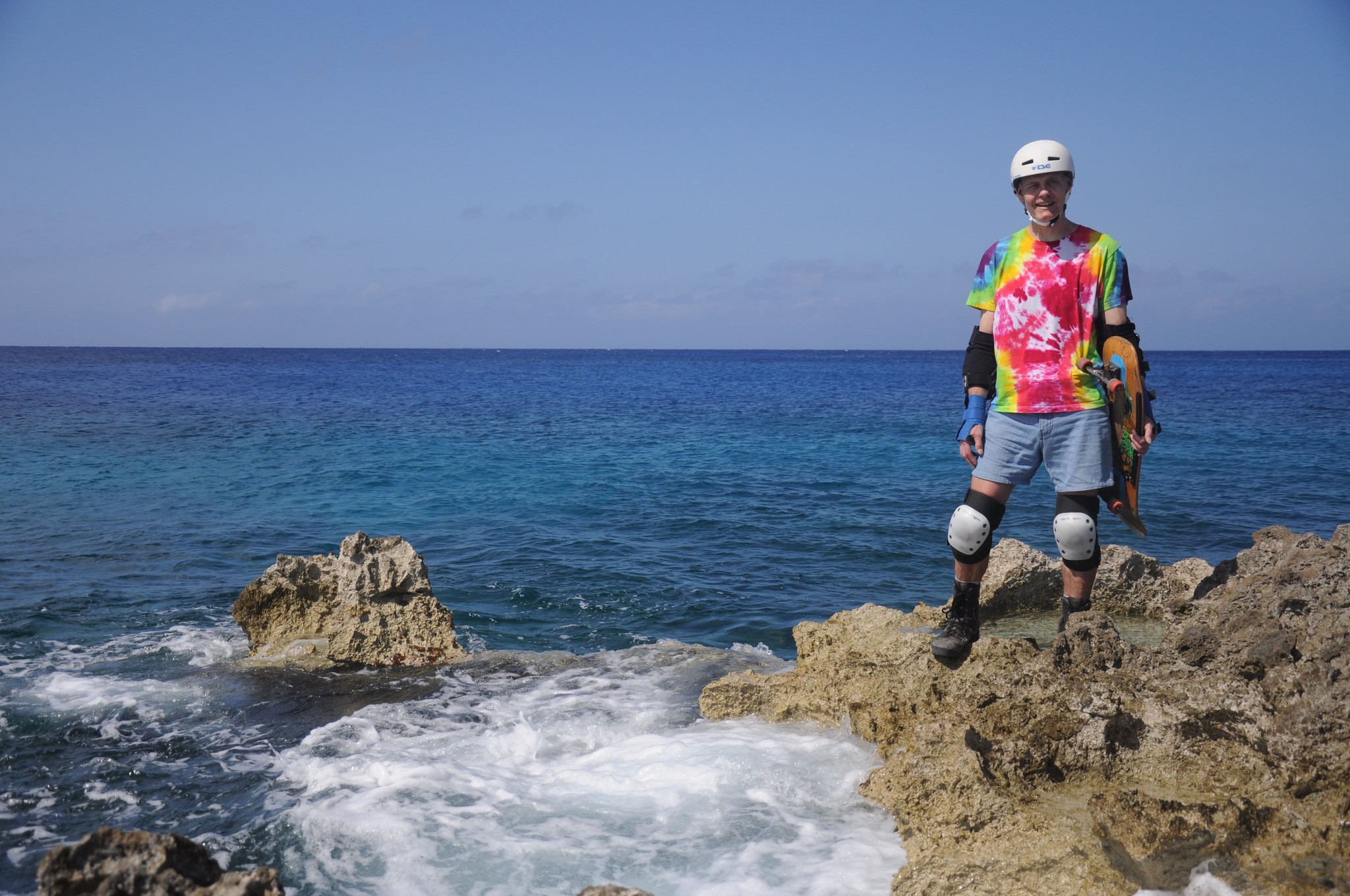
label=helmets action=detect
[1010,139,1075,189]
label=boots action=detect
[1056,597,1091,634]
[932,581,980,657]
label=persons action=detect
[931,138,1157,660]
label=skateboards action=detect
[1074,334,1148,541]
